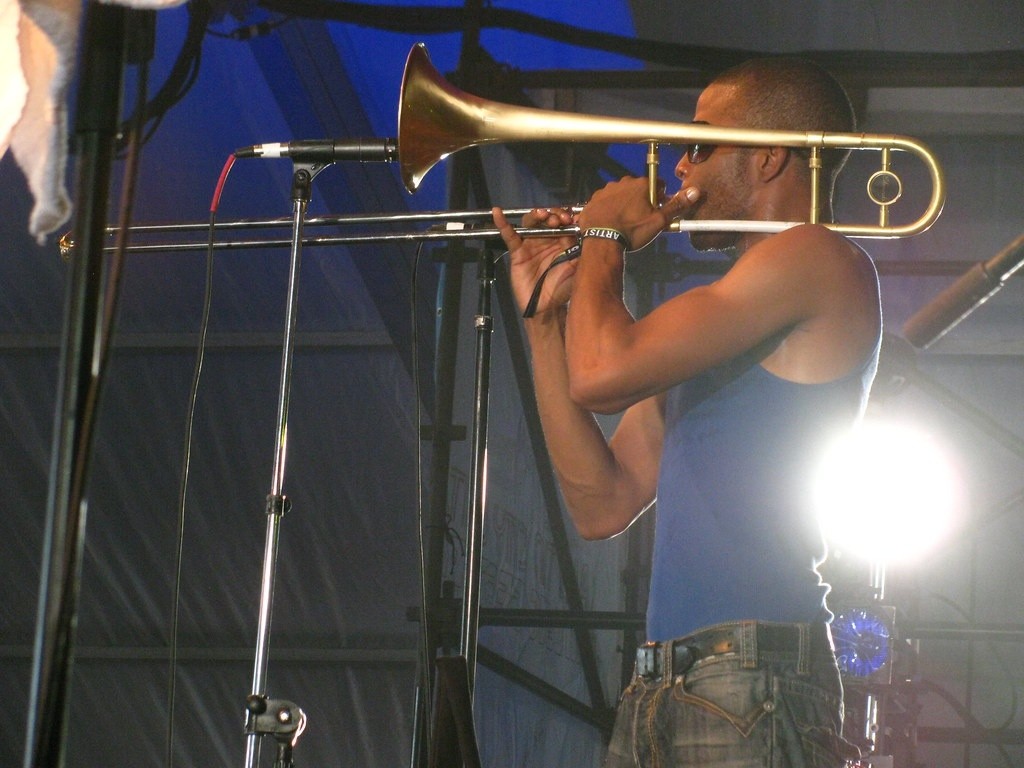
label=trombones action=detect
[54,41,948,261]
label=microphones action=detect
[233,135,398,162]
[429,221,521,231]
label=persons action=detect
[497,61,885,768]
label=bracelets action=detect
[580,228,630,250]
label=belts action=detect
[635,623,835,678]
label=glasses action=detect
[688,121,717,164]
